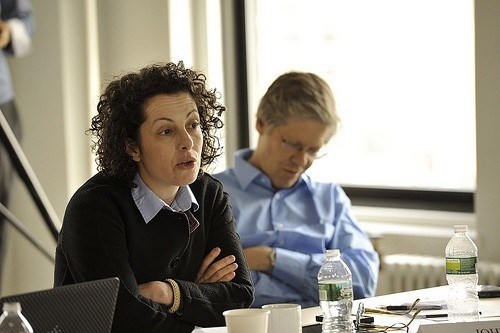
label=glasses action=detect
[275,125,330,160]
[356,298,421,330]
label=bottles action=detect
[317,248,356,333]
[0,302,33,333]
[445,225,480,323]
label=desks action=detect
[194,283,500,333]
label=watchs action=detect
[268,248,276,269]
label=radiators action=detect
[381,252,500,295]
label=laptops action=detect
[0,277,120,333]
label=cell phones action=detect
[316,314,374,324]
[476,285,500,298]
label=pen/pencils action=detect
[380,305,443,310]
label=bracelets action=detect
[165,279,180,314]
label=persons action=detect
[0,0,37,240]
[53,60,255,333]
[209,71,381,309]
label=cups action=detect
[222,309,271,333]
[262,304,302,333]
